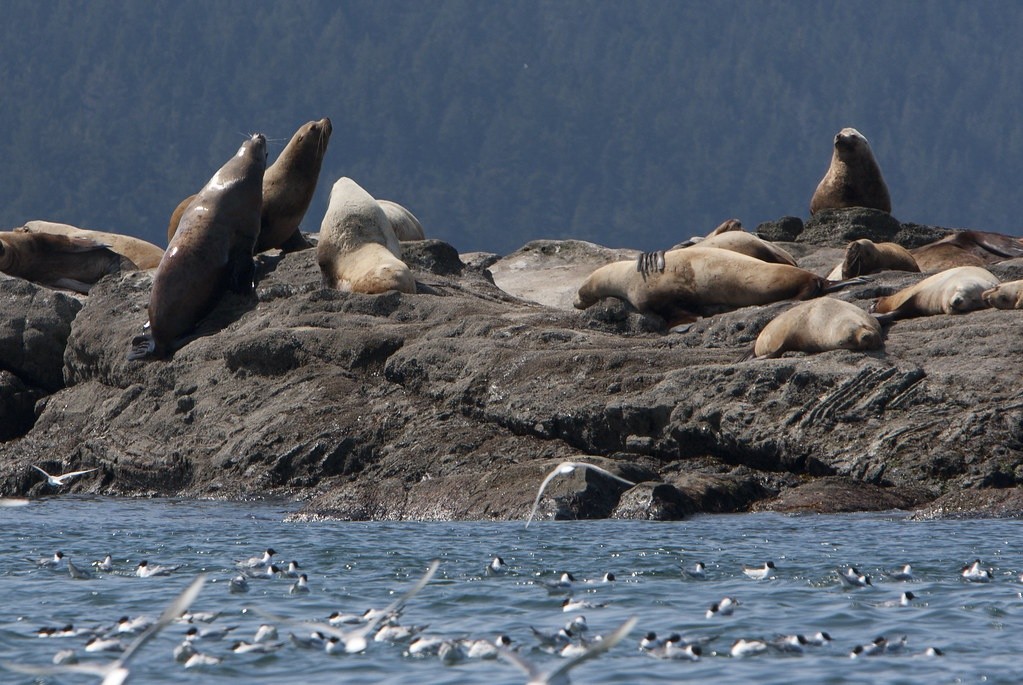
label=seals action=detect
[732,297,883,363]
[572,217,878,324]
[129,133,266,361]
[824,228,1023,318]
[168,116,332,258]
[316,176,425,296]
[0,220,167,294]
[810,127,891,214]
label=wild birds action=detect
[224,548,312,596]
[728,633,944,660]
[27,550,184,581]
[525,462,637,526]
[0,574,284,685]
[639,630,706,664]
[289,560,638,685]
[673,555,993,618]
[483,555,507,577]
[27,462,100,485]
